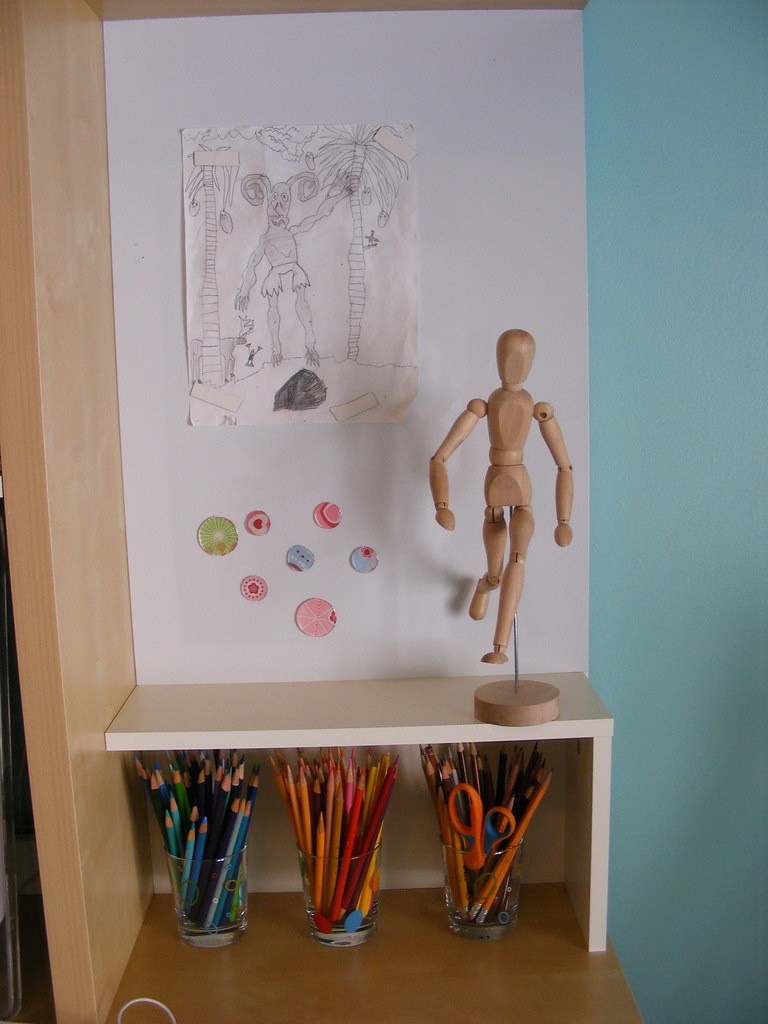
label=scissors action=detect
[447,783,518,896]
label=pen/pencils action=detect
[131,744,264,946]
[268,747,403,944]
[416,742,559,941]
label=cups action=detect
[164,846,249,949]
[298,845,382,948]
[439,835,526,941]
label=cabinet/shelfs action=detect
[0,0,650,1024]
